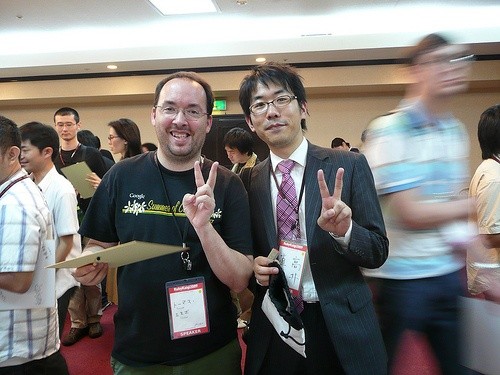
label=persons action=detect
[71,71,254,375]
[0,115,71,375]
[331,138,360,153]
[466,104,499,305]
[222,127,261,329]
[18,107,158,346]
[362,32,478,375]
[240,61,391,375]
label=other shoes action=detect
[87,322,103,338]
[61,327,89,346]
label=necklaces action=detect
[56,142,82,170]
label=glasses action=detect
[107,134,121,140]
[154,103,209,121]
[248,94,298,116]
[55,121,77,129]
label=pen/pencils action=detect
[92,256,101,267]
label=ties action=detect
[276,160,304,314]
